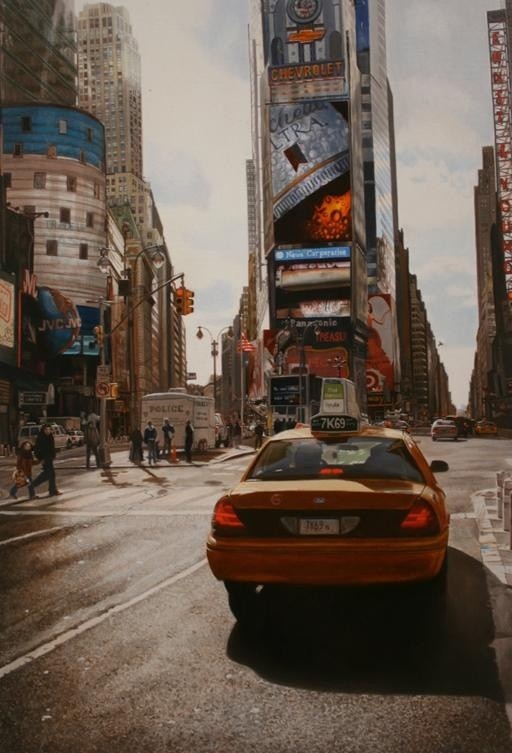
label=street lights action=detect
[95,245,167,441]
[279,314,325,424]
[195,325,236,413]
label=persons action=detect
[84,421,100,468]
[185,419,193,463]
[28,424,63,496]
[9,441,41,500]
[144,420,158,464]
[127,427,145,461]
[226,417,298,451]
[162,418,175,456]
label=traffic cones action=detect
[168,442,179,462]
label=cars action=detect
[16,414,84,452]
[376,410,500,441]
[206,376,451,620]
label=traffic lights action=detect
[185,289,194,315]
[93,323,103,347]
[175,287,186,315]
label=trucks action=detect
[140,387,216,453]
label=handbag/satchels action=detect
[13,470,30,489]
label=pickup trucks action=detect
[215,413,231,449]
